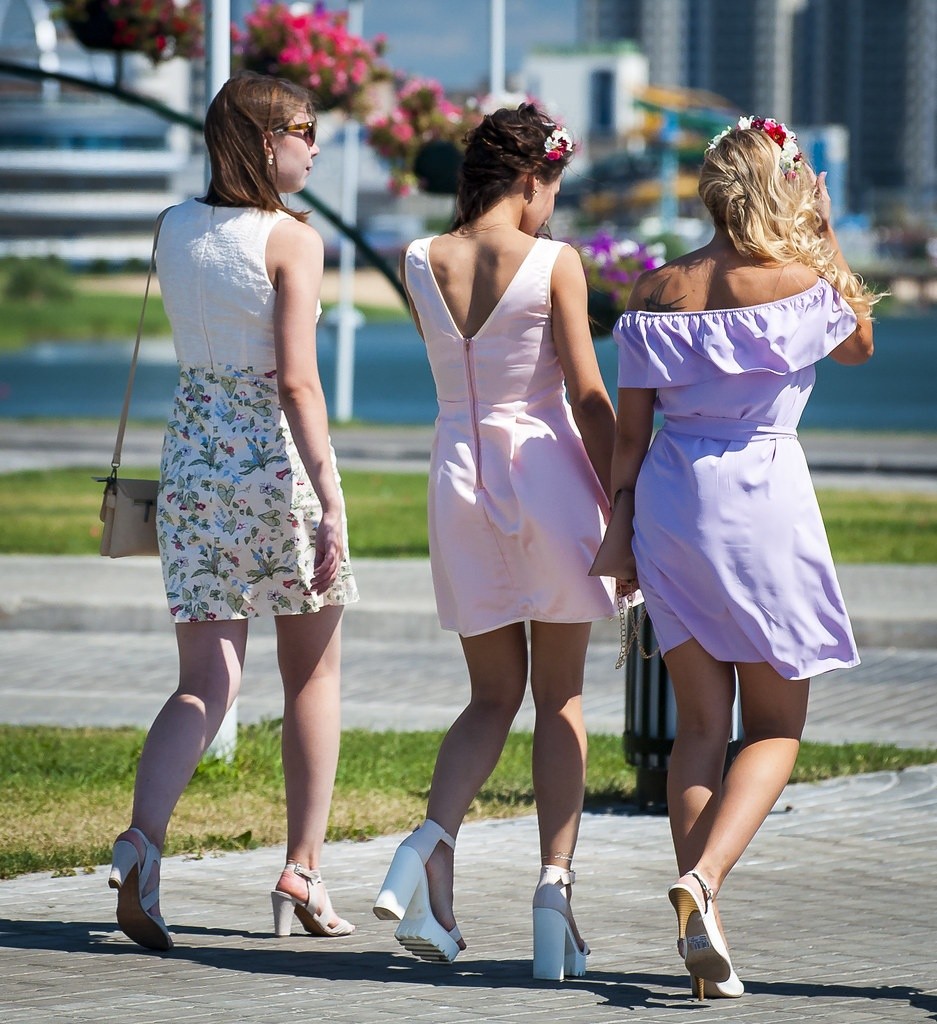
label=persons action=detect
[110,71,360,951]
[372,102,618,982]
[609,117,875,1000]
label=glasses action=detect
[273,111,316,147]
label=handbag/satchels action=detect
[99,477,164,558]
[588,488,637,582]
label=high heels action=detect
[271,864,356,937]
[374,820,467,964]
[108,828,173,951]
[691,961,745,1001]
[669,870,731,983]
[532,864,590,980]
